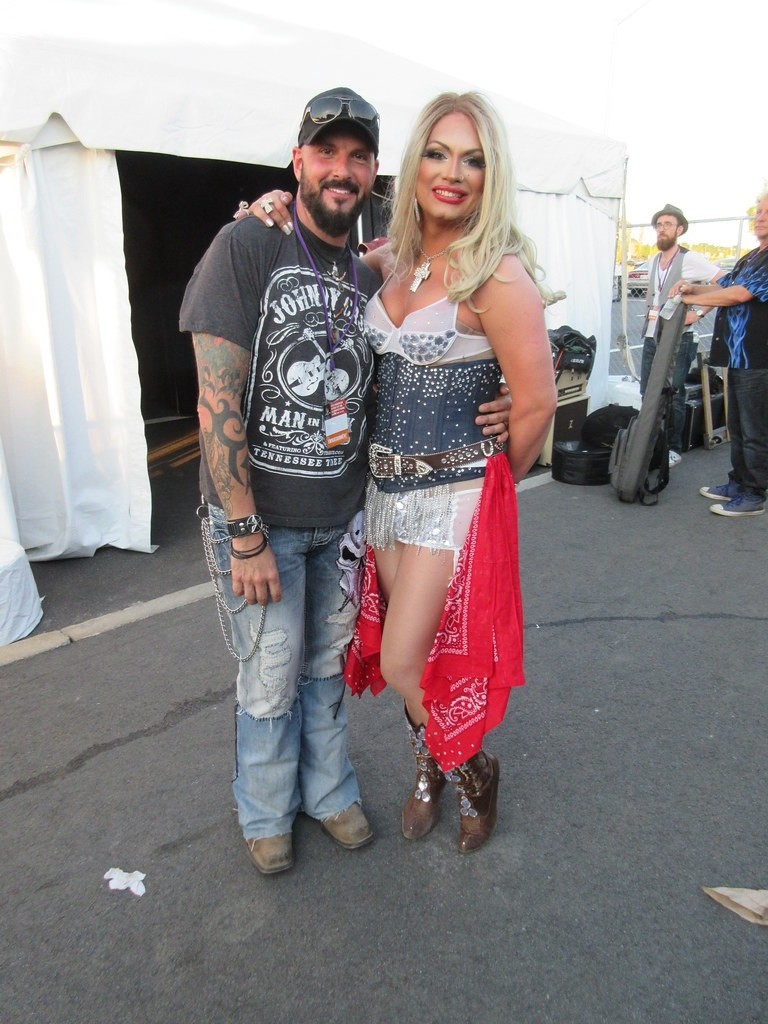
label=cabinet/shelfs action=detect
[535,394,592,468]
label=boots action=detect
[431,747,500,854]
[401,698,448,838]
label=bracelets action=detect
[231,534,267,560]
[227,513,269,539]
[695,308,705,318]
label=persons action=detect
[667,193,768,517]
[229,90,559,854]
[180,86,515,874]
[356,235,390,255]
[639,204,727,468]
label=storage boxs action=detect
[544,367,590,403]
[681,382,724,451]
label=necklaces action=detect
[409,246,444,293]
[309,243,350,293]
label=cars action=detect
[627,261,649,296]
[718,257,740,274]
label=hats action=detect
[651,204,688,236]
[298,87,379,158]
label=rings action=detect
[260,198,273,214]
[233,201,249,219]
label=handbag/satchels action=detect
[582,404,639,448]
[547,325,597,384]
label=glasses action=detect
[655,222,673,229]
[298,97,379,137]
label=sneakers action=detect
[699,480,743,501]
[709,493,765,516]
[669,450,681,467]
[246,833,294,874]
[320,802,375,850]
